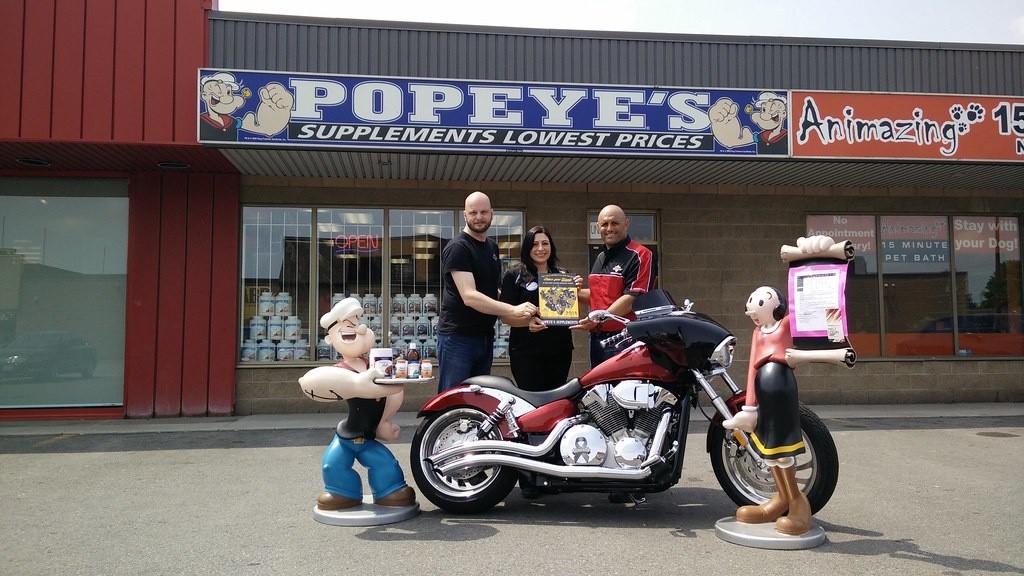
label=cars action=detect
[918,312,1024,336]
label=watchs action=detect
[596,324,602,329]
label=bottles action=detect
[268,316,284,340]
[406,343,421,379]
[285,316,301,340]
[395,360,408,380]
[294,340,310,360]
[421,360,432,378]
[259,292,275,316]
[256,339,276,361]
[317,293,440,360]
[242,340,258,361]
[250,316,266,339]
[277,340,294,361]
[276,292,292,316]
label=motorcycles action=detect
[409,287,839,517]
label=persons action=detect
[498,226,583,392]
[568,205,658,370]
[722,235,835,534]
[298,298,416,510]
[436,191,537,394]
